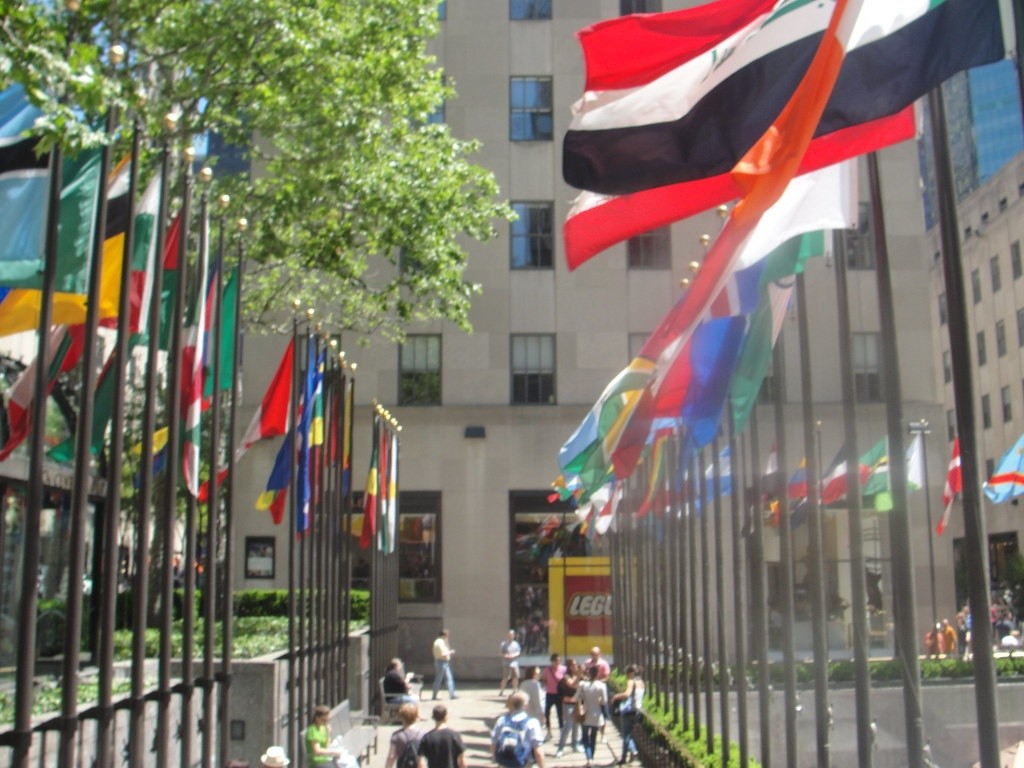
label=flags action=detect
[1,68,399,560]
[549,0,1024,542]
[513,516,585,580]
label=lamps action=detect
[464,425,486,445]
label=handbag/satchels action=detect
[570,701,586,724]
[622,703,646,724]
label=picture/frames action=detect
[244,536,276,579]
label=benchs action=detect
[379,673,423,726]
[299,698,380,768]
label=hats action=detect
[260,745,289,768]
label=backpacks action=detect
[395,729,423,768]
[494,713,535,768]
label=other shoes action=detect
[617,759,626,765]
[601,734,607,744]
[542,733,553,743]
[573,747,584,753]
[629,751,638,762]
[555,750,563,758]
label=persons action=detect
[378,626,646,768]
[925,578,1024,661]
[261,746,291,768]
[514,586,550,655]
[305,705,361,768]
